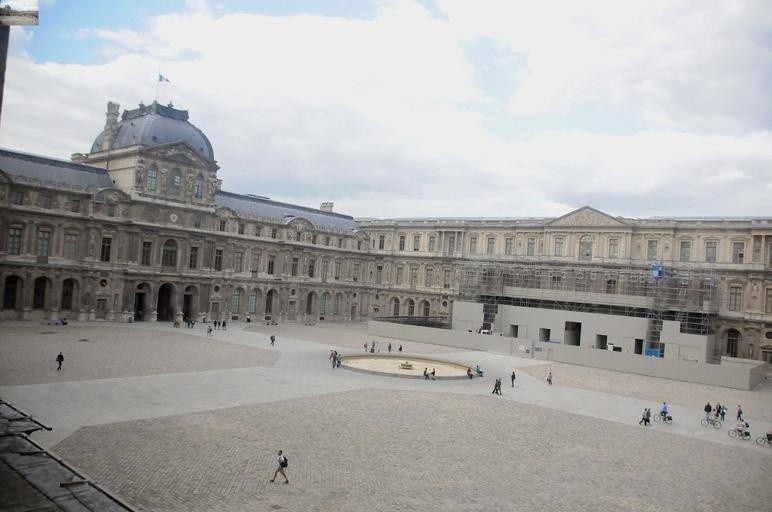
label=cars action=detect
[480,330,492,335]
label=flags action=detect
[159,74,169,81]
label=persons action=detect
[364,341,403,353]
[424,368,435,380]
[639,407,650,426]
[511,371,515,387]
[702,401,772,451]
[55,351,64,370]
[492,377,502,396]
[270,333,275,345]
[186,316,226,337]
[476,365,483,376]
[329,349,341,369]
[547,372,553,384]
[659,401,668,421]
[467,367,473,380]
[270,450,289,484]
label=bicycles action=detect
[653,413,673,425]
[700,416,722,429]
[755,436,772,447]
[728,425,752,441]
